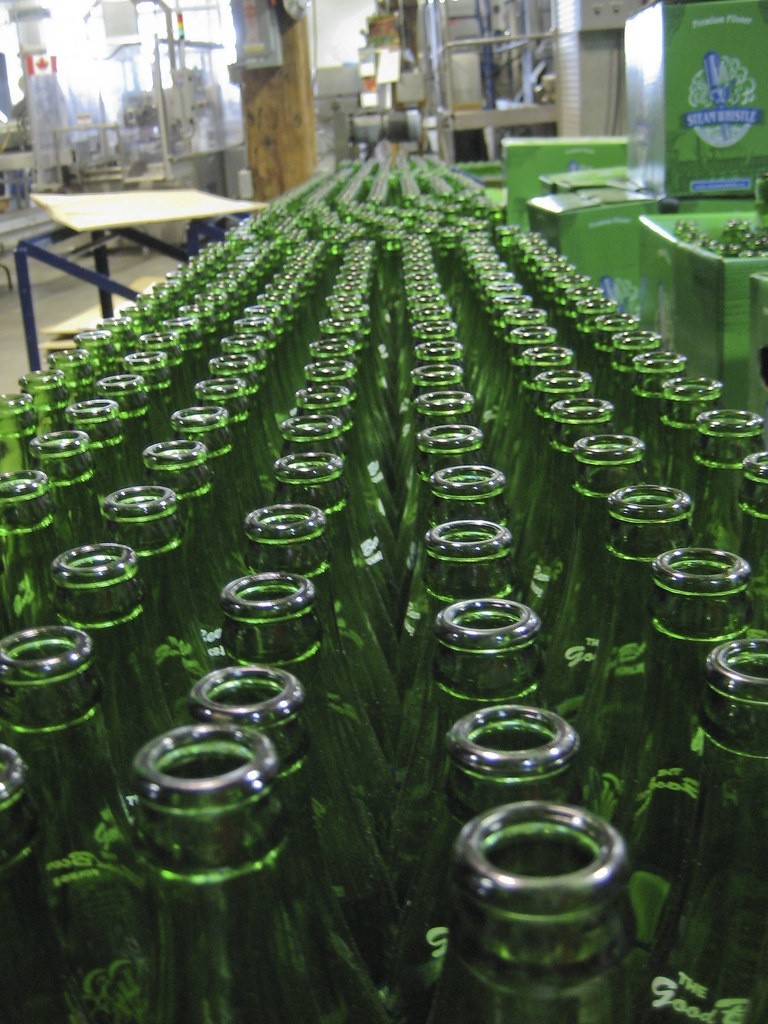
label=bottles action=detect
[0,136,768,1023]
[600,276,617,304]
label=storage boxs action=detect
[503,0,768,454]
